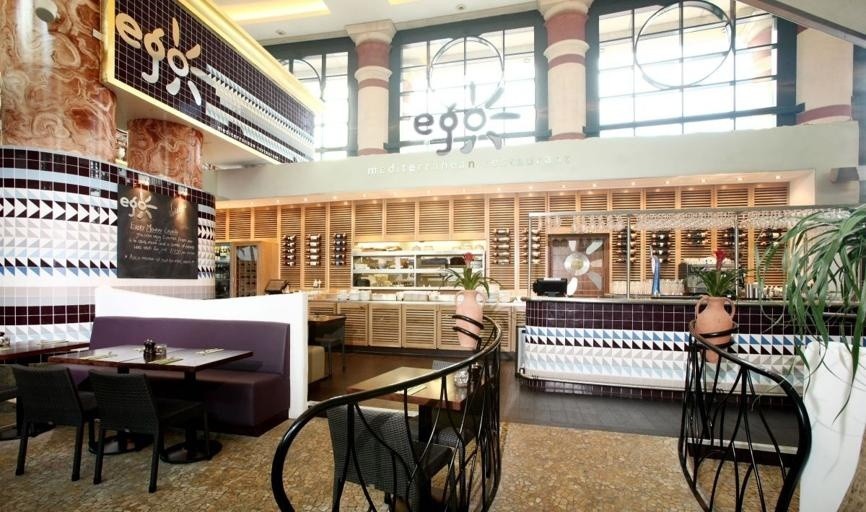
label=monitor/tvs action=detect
[266,278,288,291]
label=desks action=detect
[345,365,491,511]
[307,314,347,376]
[48,344,253,465]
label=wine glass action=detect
[544,208,849,233]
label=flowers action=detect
[692,250,756,296]
[444,253,505,299]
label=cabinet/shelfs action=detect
[350,249,486,292]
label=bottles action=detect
[763,285,783,297]
[616,232,785,262]
[491,228,541,265]
[281,233,346,267]
[143,339,166,361]
[215,245,229,281]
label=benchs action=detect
[63,317,290,437]
[308,344,325,402]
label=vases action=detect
[695,296,736,363]
[453,290,485,350]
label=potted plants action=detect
[758,204,866,512]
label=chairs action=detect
[327,406,458,512]
[312,314,348,379]
[424,359,491,488]
[10,364,212,493]
